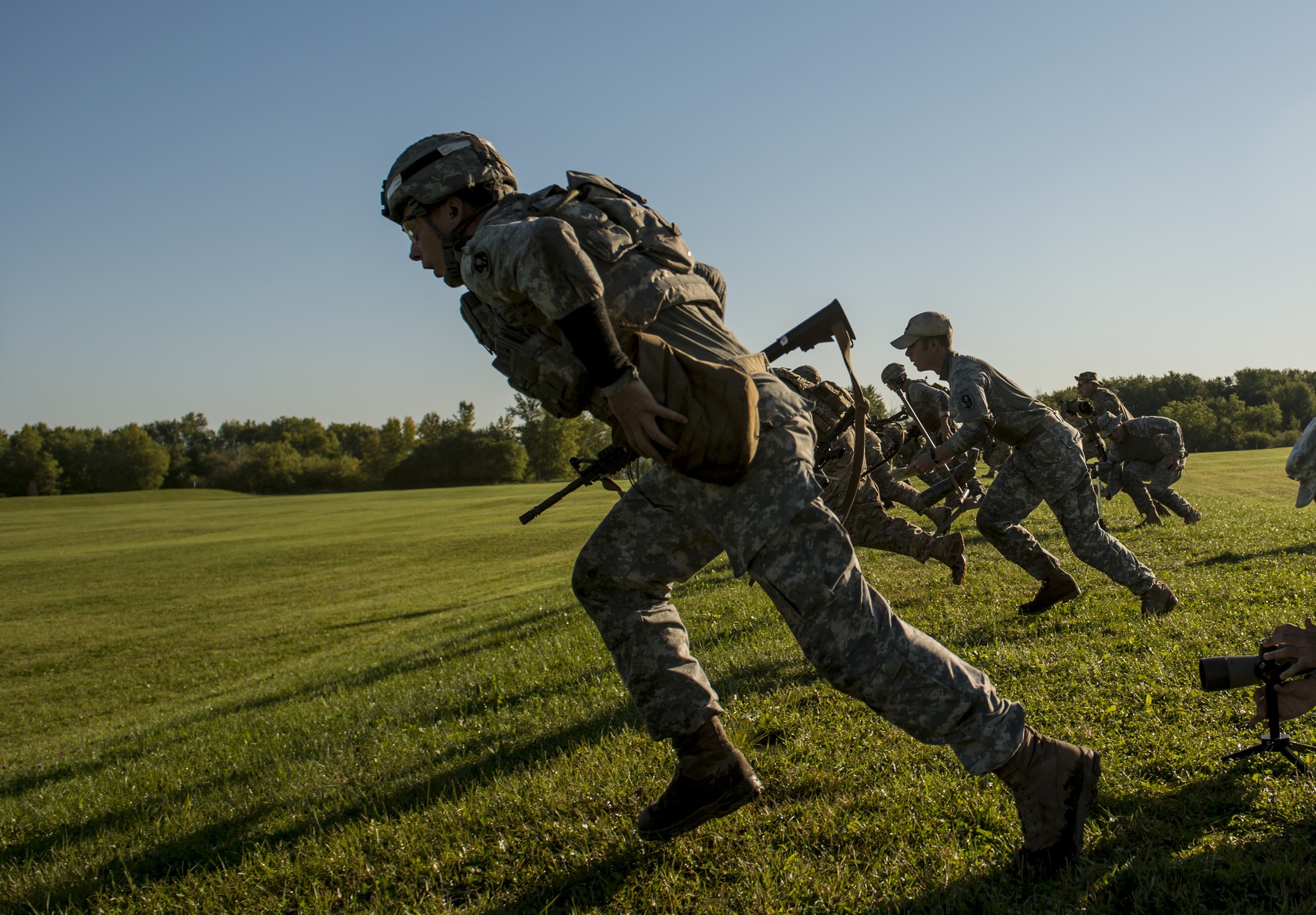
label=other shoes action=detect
[883,504,895,509]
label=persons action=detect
[1063,409,1108,465]
[1074,371,1151,497]
[792,364,958,540]
[868,417,963,512]
[880,361,988,509]
[377,127,1105,870]
[1247,411,1316,728]
[889,307,1182,622]
[980,440,1014,479]
[767,360,968,585]
[977,436,998,470]
[1090,410,1203,531]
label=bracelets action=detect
[929,448,941,465]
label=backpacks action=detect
[524,169,696,275]
[815,380,854,418]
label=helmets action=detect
[792,365,822,383]
[881,363,905,384]
[381,130,518,224]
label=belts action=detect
[1018,409,1062,447]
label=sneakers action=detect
[1141,579,1177,621]
[925,505,952,539]
[1134,503,1201,529]
[992,722,1101,867]
[943,491,962,510]
[1017,568,1081,615]
[972,494,987,502]
[930,532,967,586]
[637,716,765,841]
[981,473,995,479]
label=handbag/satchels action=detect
[627,330,759,484]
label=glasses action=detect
[886,380,893,388]
[1077,381,1088,387]
[401,215,424,242]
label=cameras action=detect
[1200,638,1315,691]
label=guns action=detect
[805,403,855,492]
[515,299,855,537]
[1083,416,1113,473]
[870,403,907,434]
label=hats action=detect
[1074,371,1105,385]
[1285,416,1316,508]
[890,311,953,350]
[1097,411,1124,439]
